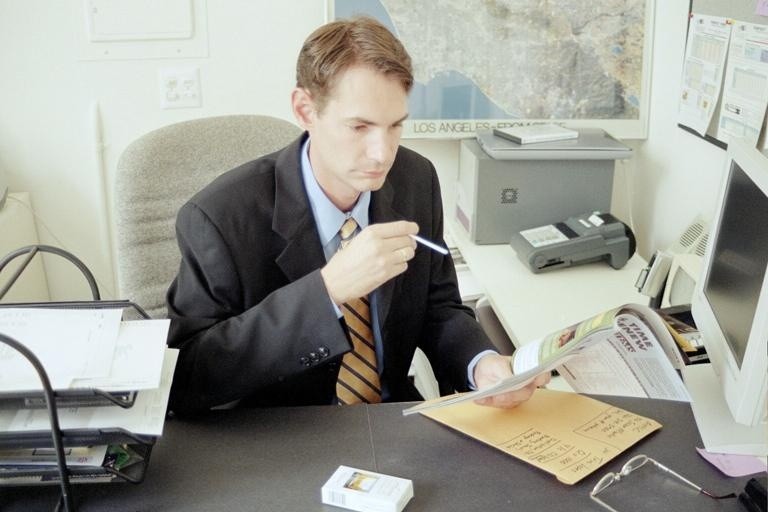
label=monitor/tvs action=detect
[678,134,768,454]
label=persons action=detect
[167,18,551,423]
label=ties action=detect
[337,219,382,407]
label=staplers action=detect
[739,477,768,512]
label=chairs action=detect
[111,114,303,402]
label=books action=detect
[401,303,695,416]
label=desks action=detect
[0,393,766,512]
[448,221,652,350]
